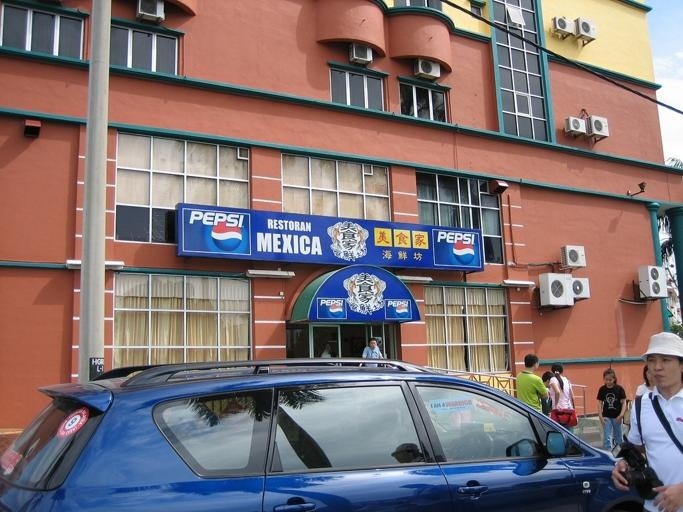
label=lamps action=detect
[626,181,646,197]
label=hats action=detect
[640,332,683,362]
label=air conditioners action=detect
[573,16,596,40]
[551,15,575,36]
[348,42,373,65]
[565,116,586,135]
[413,56,440,82]
[538,273,574,306]
[560,245,586,268]
[572,277,590,299]
[638,265,668,299]
[585,114,609,138]
[135,0,165,21]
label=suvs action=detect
[0,355,662,512]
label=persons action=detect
[515,354,576,436]
[611,333,682,512]
[358,338,385,368]
[635,365,654,395]
[596,369,627,450]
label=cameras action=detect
[612,441,664,499]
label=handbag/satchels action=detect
[550,408,578,427]
